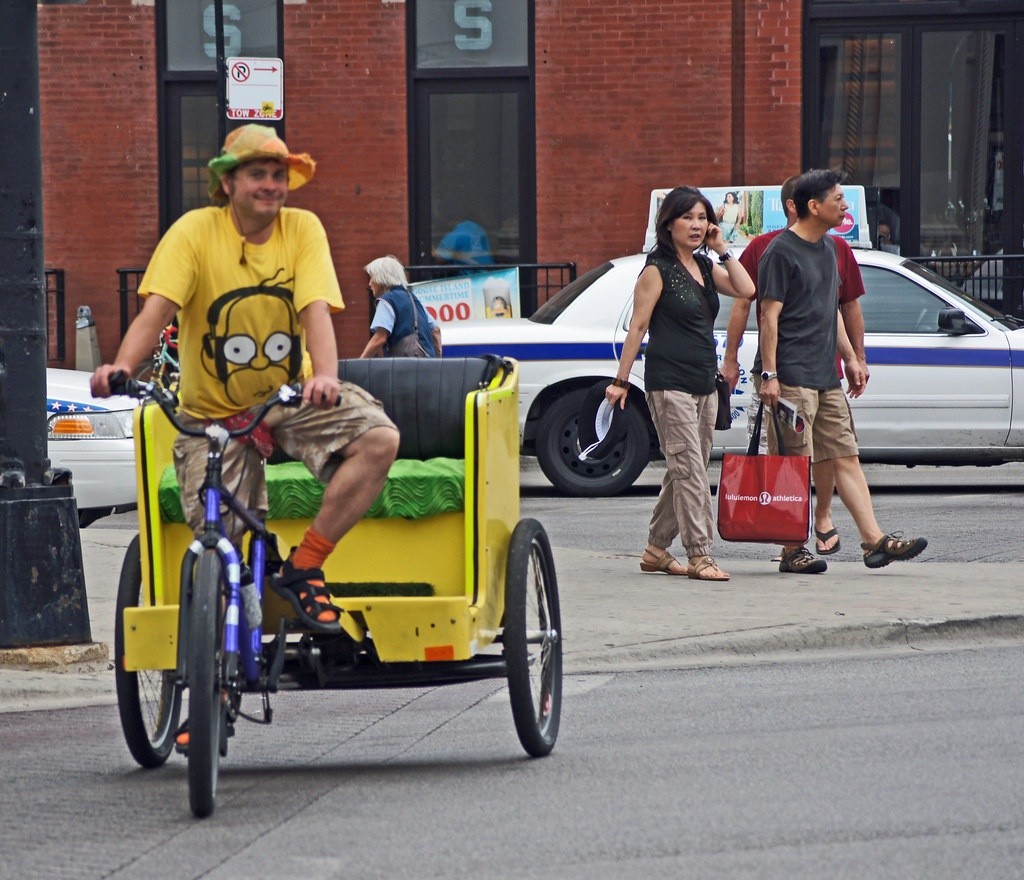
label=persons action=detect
[604,165,929,583]
[432,202,493,274]
[362,253,440,358]
[91,123,399,754]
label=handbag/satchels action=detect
[383,335,430,359]
[714,371,731,431]
[716,401,813,547]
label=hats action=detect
[207,122,315,200]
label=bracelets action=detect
[611,377,631,390]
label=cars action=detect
[46,368,152,526]
[409,237,1024,493]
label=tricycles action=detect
[90,353,565,818]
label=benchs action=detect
[160,355,501,524]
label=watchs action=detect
[761,369,778,380]
[717,248,733,262]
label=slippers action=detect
[814,522,841,555]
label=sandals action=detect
[270,560,344,633]
[778,545,828,574]
[861,530,928,569]
[639,546,690,575]
[173,719,192,755]
[686,556,731,581]
